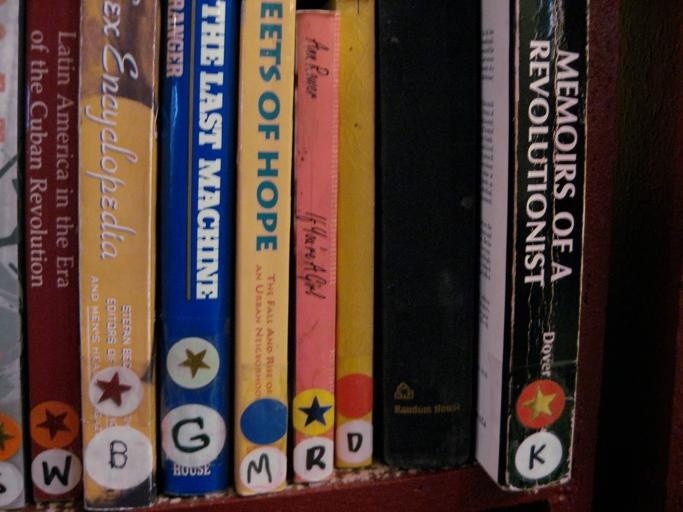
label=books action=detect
[0,0,590,511]
